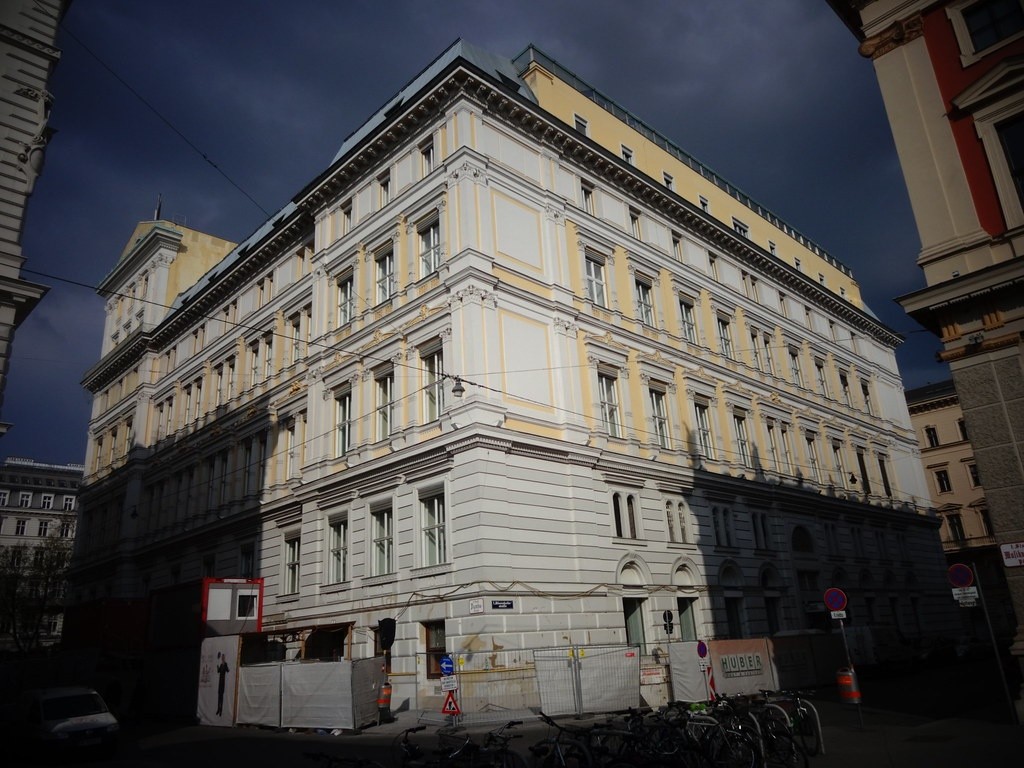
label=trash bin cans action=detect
[835,665,861,705]
[376,682,392,710]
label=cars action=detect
[27,685,121,753]
[953,635,986,655]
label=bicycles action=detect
[389,688,827,768]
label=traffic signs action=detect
[440,656,453,674]
